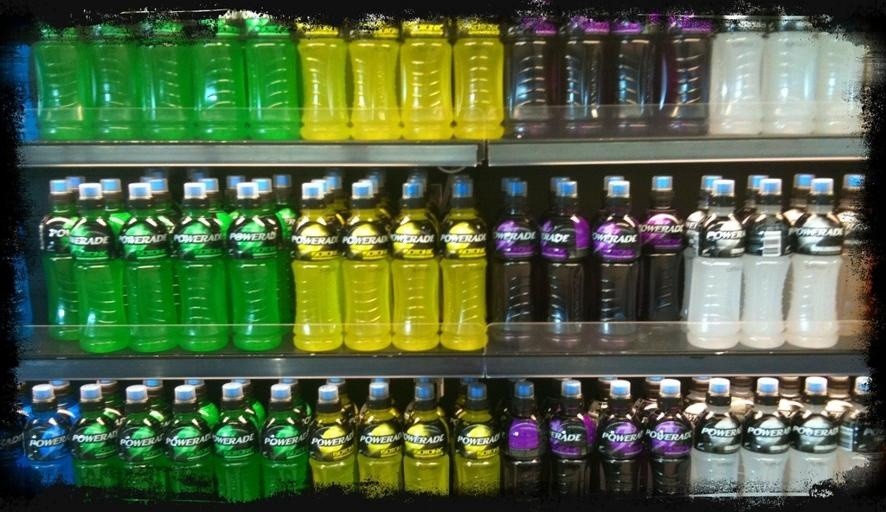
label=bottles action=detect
[2,11,880,147]
[3,374,879,512]
[6,162,878,362]
